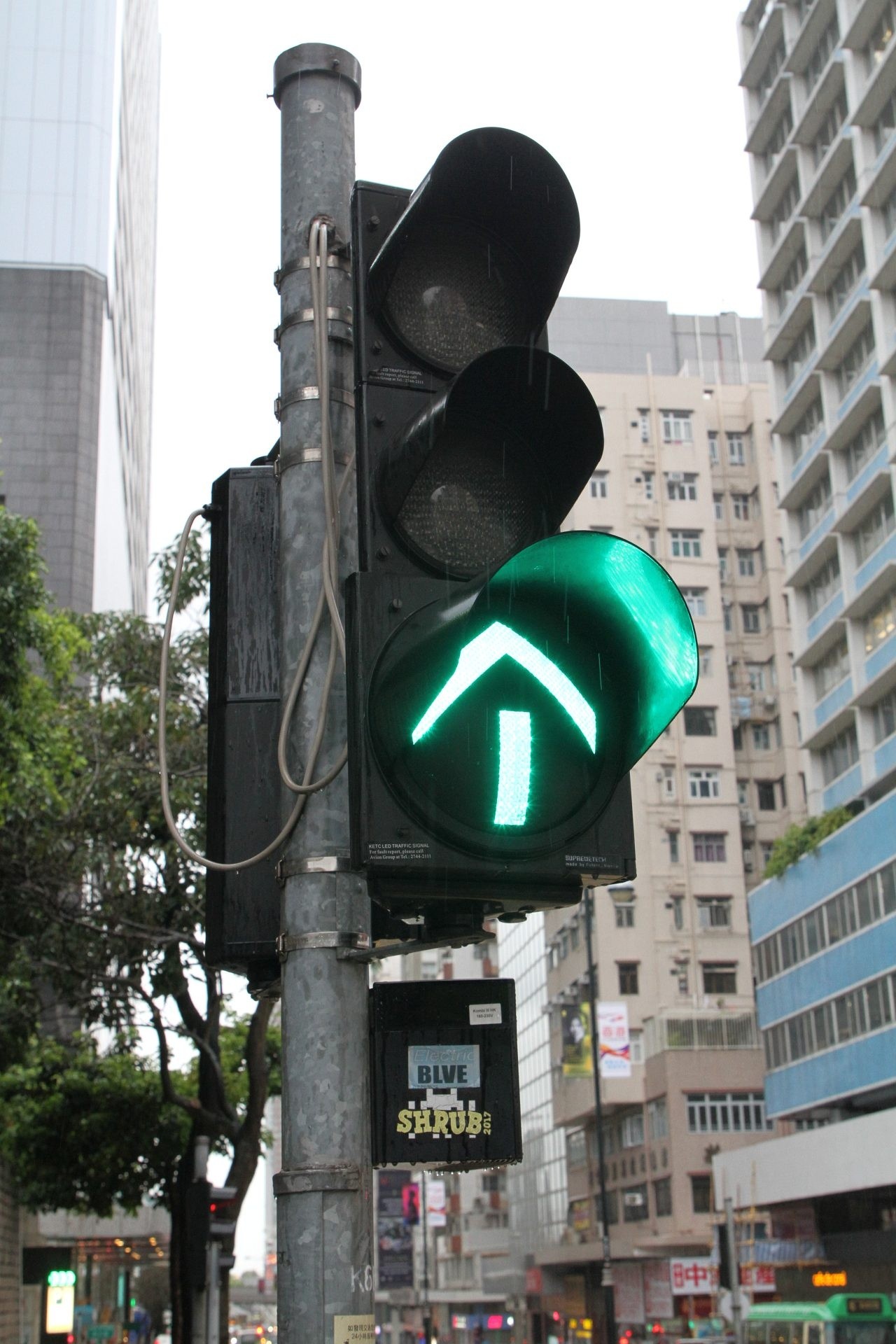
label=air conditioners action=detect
[625,1192,644,1207]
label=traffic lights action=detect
[199,1186,236,1247]
[348,127,696,890]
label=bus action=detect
[742,1294,896,1344]
